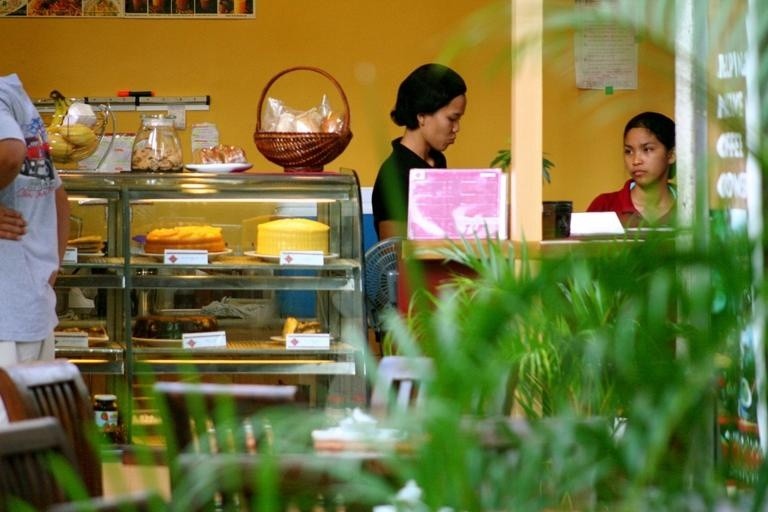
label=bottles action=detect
[129,112,184,173]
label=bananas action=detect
[44,90,73,128]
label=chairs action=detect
[1,354,522,510]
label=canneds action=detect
[93,394,119,428]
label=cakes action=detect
[256,218,331,255]
[68,235,102,253]
[133,316,217,339]
[144,224,225,253]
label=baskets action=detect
[253,65,353,174]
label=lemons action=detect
[48,134,74,158]
[60,124,97,146]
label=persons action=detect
[586,112,675,228]
[372,64,466,255]
[0,73,70,367]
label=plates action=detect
[130,335,183,348]
[130,246,234,265]
[310,423,409,444]
[89,332,110,344]
[243,249,340,264]
[77,251,106,258]
[269,335,336,344]
[183,162,255,174]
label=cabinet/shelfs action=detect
[52,168,368,464]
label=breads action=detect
[196,144,247,163]
[280,314,320,338]
[264,93,344,132]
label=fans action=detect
[353,235,402,331]
